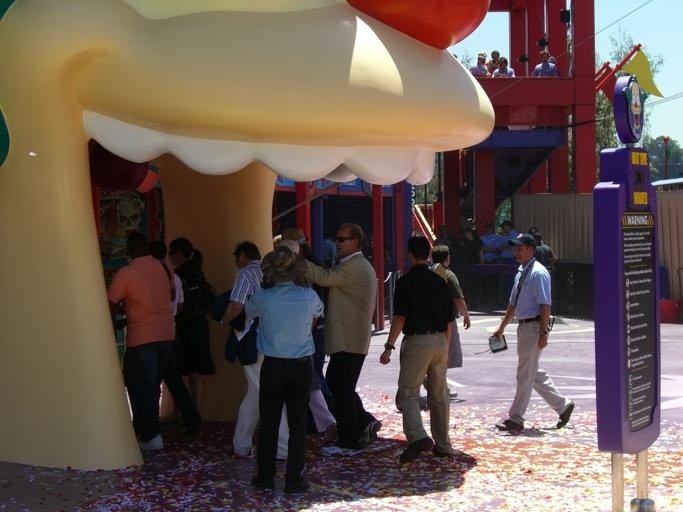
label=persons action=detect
[282,227,334,410]
[491,57,515,78]
[463,227,484,309]
[168,237,208,416]
[486,51,500,73]
[493,233,575,430]
[243,247,325,495]
[108,231,176,451]
[323,231,336,268]
[149,240,202,437]
[531,51,560,76]
[480,222,497,265]
[379,236,459,463]
[465,216,473,224]
[279,240,338,443]
[434,225,449,245]
[305,222,382,457]
[220,242,289,463]
[470,56,491,76]
[532,232,554,269]
[496,220,518,305]
[424,244,470,397]
[529,227,537,233]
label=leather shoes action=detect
[250,477,274,491]
[286,481,310,491]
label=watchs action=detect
[385,343,395,350]
[220,320,224,325]
[539,330,549,336]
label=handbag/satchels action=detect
[210,291,245,331]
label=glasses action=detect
[333,237,356,243]
[168,249,176,256]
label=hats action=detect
[273,227,311,256]
[260,245,308,283]
[508,233,538,247]
[484,222,493,229]
[499,221,511,227]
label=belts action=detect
[410,329,441,335]
[519,318,537,324]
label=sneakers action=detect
[548,315,555,330]
[495,420,524,430]
[400,438,434,463]
[319,445,364,458]
[320,425,341,447]
[362,421,382,447]
[556,401,575,429]
[136,435,165,452]
